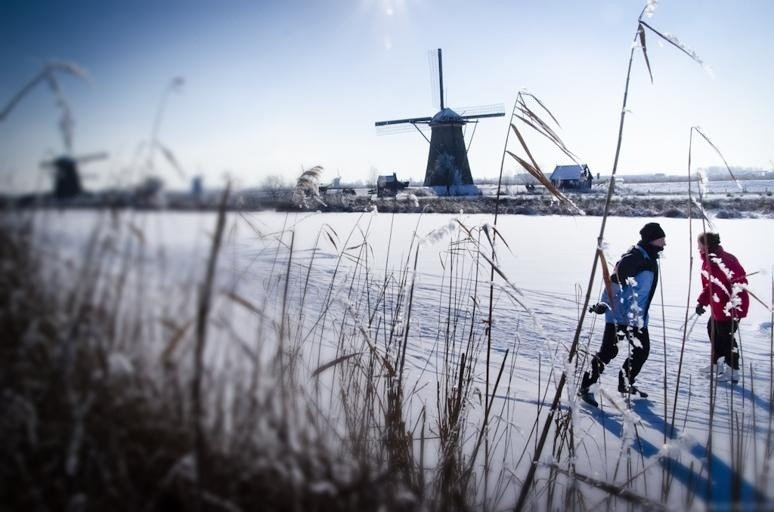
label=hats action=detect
[639,223,665,242]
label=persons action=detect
[577,223,666,407]
[695,233,749,382]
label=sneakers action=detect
[618,383,648,397]
[577,387,598,407]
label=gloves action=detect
[696,304,706,316]
[729,319,738,333]
[589,302,608,315]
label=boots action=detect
[715,366,739,381]
[700,357,725,374]
